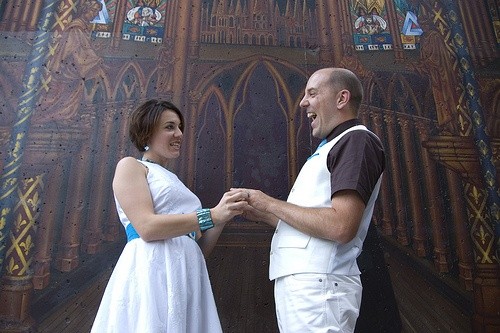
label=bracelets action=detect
[196,207,214,232]
[89,99,249,332]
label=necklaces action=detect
[143,157,174,172]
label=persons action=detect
[229,68,386,333]
